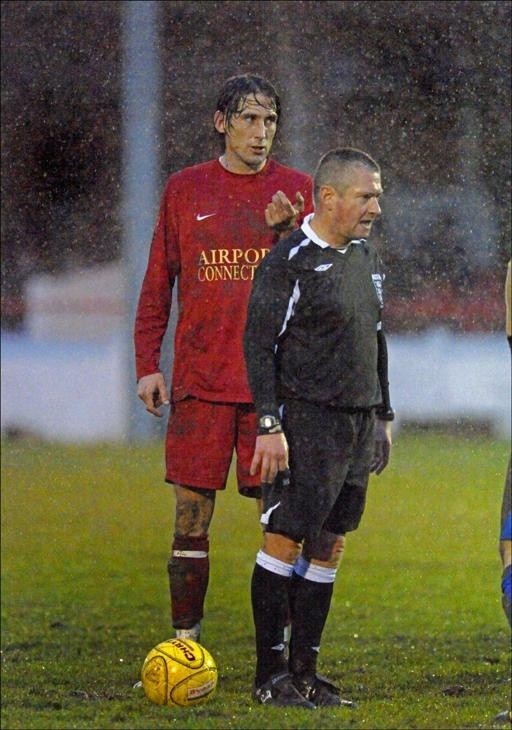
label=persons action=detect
[498,260,511,627]
[243,148,394,711]
[133,73,316,688]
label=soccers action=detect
[141,637,216,707]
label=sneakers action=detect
[251,673,318,711]
[291,671,356,710]
